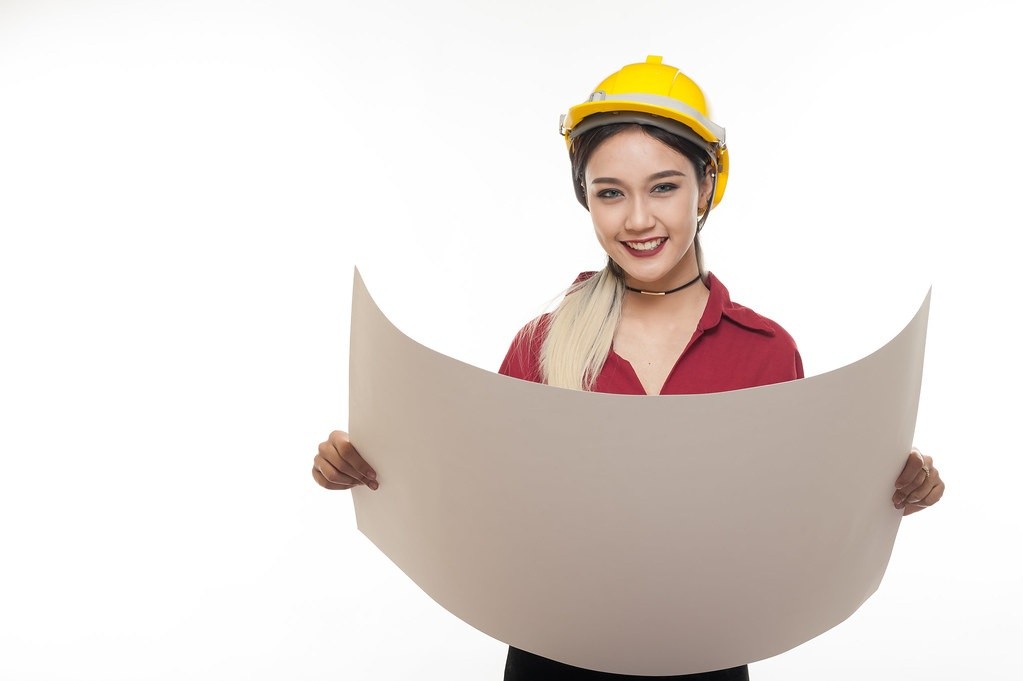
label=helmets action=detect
[557,53,731,213]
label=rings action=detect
[921,467,930,477]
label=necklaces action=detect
[623,275,701,295]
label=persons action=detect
[311,54,945,680]
